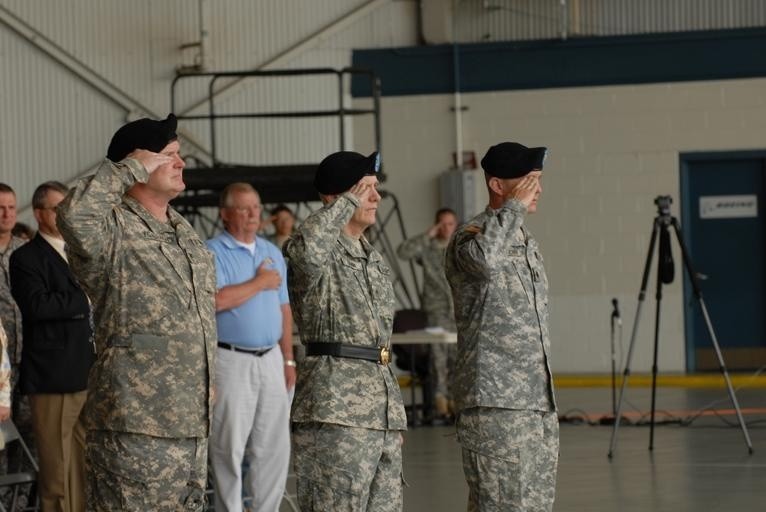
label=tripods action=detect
[605,190,756,464]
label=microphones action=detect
[610,296,622,319]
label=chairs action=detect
[0,417,41,512]
[392,309,429,425]
[206,465,300,512]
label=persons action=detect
[445,142,559,512]
[286,151,406,512]
[10,182,89,511]
[264,206,297,249]
[0,184,31,283]
[204,182,295,512]
[397,209,459,424]
[56,112,217,511]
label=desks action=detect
[390,332,458,424]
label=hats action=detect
[106,113,178,163]
[480,142,547,180]
[314,150,379,196]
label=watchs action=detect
[285,361,300,366]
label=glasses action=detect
[222,203,265,214]
[33,205,61,214]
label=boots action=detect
[449,399,458,414]
[435,397,447,418]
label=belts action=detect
[216,340,276,357]
[304,342,395,364]
